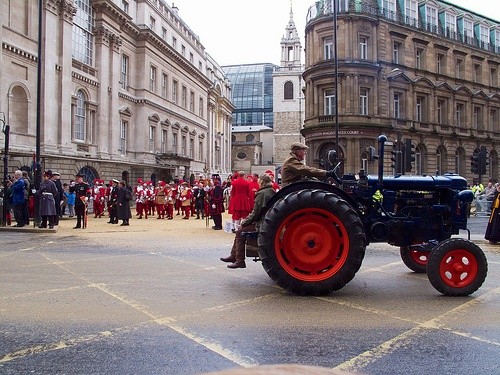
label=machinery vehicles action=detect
[239,136,489,298]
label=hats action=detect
[52,172,60,176]
[76,174,83,178]
[181,182,188,186]
[211,173,220,180]
[265,169,274,177]
[41,169,53,178]
[112,179,120,183]
[259,173,271,181]
[291,142,309,150]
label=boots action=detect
[220,236,237,264]
[227,237,246,269]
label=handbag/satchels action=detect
[124,191,131,201]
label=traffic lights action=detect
[390,151,396,168]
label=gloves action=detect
[326,170,335,177]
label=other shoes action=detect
[39,225,47,228]
[49,226,54,229]
[212,225,222,230]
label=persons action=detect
[220,173,276,268]
[138,182,152,219]
[179,182,191,219]
[35,170,58,229]
[193,183,207,220]
[115,180,132,226]
[281,141,335,188]
[107,180,119,224]
[7,169,25,227]
[0,171,131,228]
[211,175,225,231]
[91,179,104,218]
[69,175,90,229]
[134,169,281,228]
[133,178,144,215]
[153,181,169,219]
[167,181,176,218]
[473,182,500,244]
[228,171,249,232]
